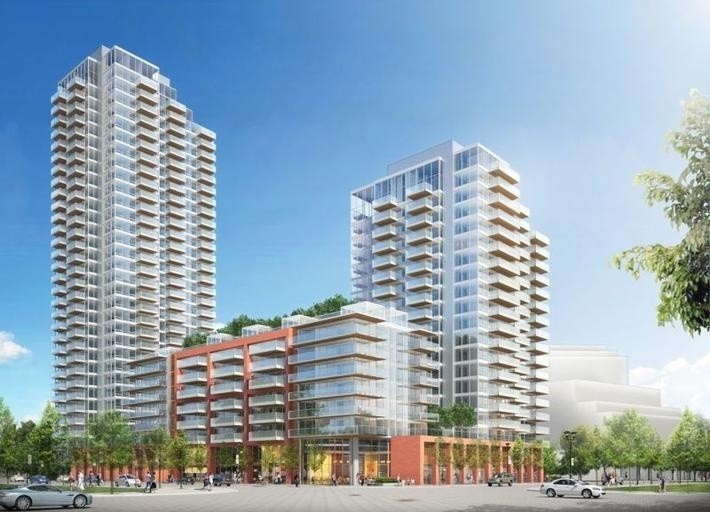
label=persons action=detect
[145,471,156,489]
[202,469,242,489]
[253,468,300,487]
[311,472,369,487]
[68,471,75,489]
[97,472,102,486]
[89,470,95,487]
[77,470,85,490]
[168,472,197,485]
[656,472,666,493]
[394,470,416,486]
